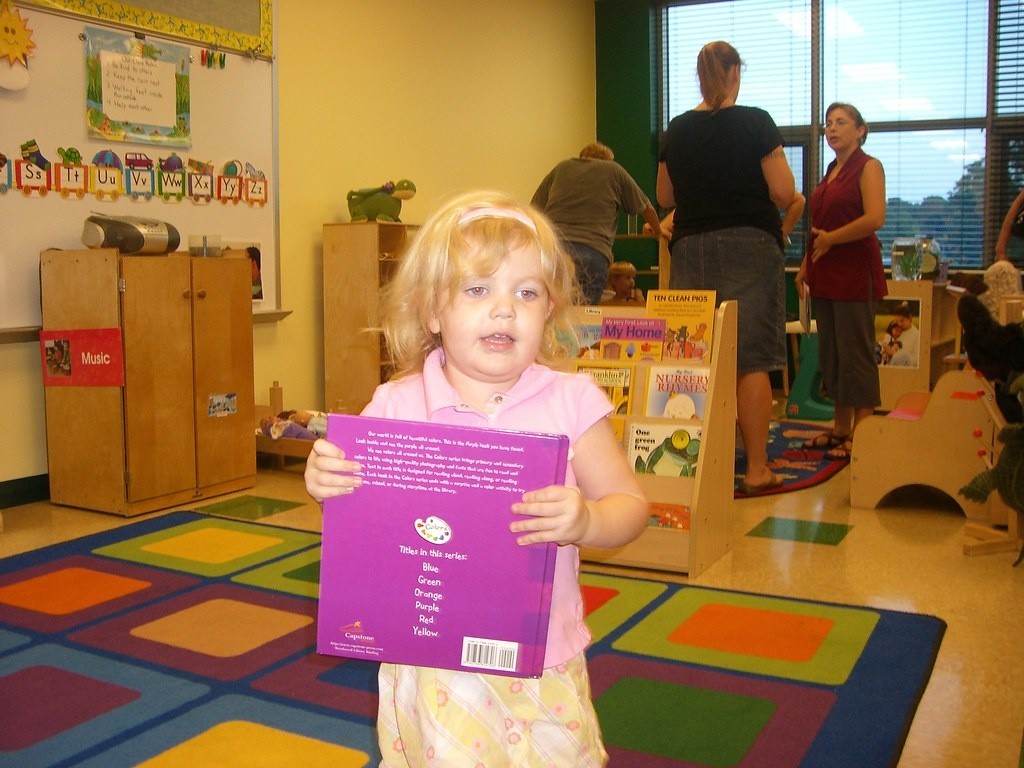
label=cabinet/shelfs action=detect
[875,280,967,411]
[40,246,257,517]
[323,221,421,416]
[614,234,671,290]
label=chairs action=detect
[849,362,995,519]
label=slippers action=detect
[739,471,783,493]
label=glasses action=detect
[824,120,855,130]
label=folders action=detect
[798,281,811,337]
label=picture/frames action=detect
[574,362,636,414]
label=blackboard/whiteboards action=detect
[0,0,293,344]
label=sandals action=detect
[801,428,853,461]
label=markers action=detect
[203,236,207,257]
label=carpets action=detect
[0,509,948,768]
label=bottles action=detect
[917,232,941,282]
[269,380,282,412]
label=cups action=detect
[940,261,949,281]
[628,214,637,234]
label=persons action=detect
[657,40,795,490]
[304,189,649,767]
[797,104,885,462]
[879,307,919,365]
[531,146,805,307]
